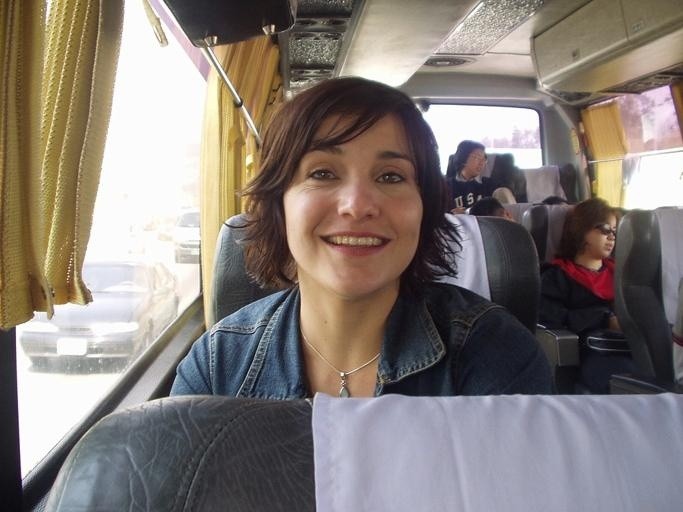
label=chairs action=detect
[213,211,541,334]
[39,392,683,511]
[530,201,600,374]
[609,207,683,392]
[449,158,577,220]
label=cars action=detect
[19,255,178,368]
[174,207,201,261]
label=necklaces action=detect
[296,326,382,399]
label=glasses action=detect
[592,222,616,236]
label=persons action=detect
[624,92,659,152]
[167,75,556,398]
[443,140,489,215]
[468,196,515,221]
[537,196,637,393]
